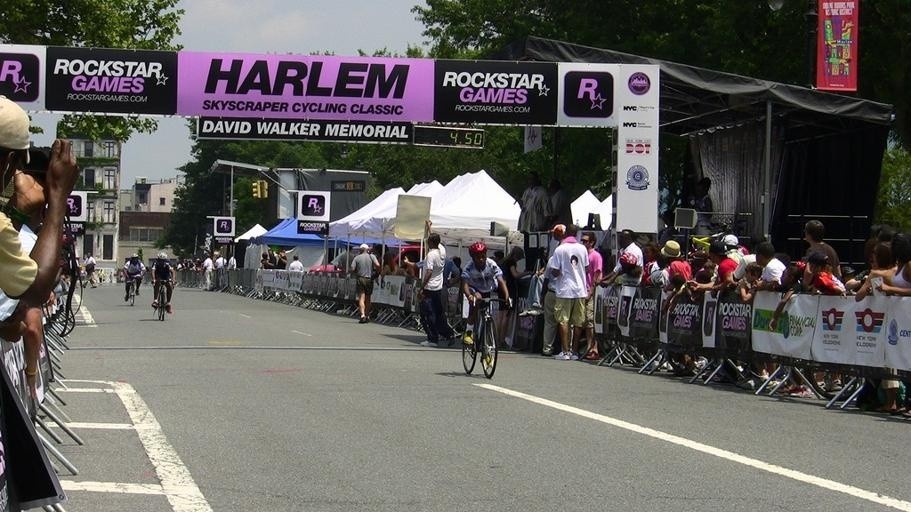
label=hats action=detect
[550,224,566,236]
[0,95,31,165]
[802,252,830,264]
[360,244,369,249]
[661,240,682,258]
[721,234,738,247]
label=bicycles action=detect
[125,274,138,304]
[151,280,172,321]
[459,290,508,379]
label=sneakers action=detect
[496,340,509,350]
[152,302,158,309]
[359,317,369,323]
[485,354,493,366]
[464,331,473,345]
[518,310,540,317]
[555,349,843,400]
[421,340,438,347]
[166,305,172,313]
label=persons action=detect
[382,220,463,341]
[350,243,381,323]
[82,252,98,288]
[338,245,355,269]
[260,249,305,270]
[0,95,81,412]
[461,240,509,368]
[122,253,146,302]
[150,252,176,315]
[492,213,911,416]
[177,250,236,291]
[97,269,105,283]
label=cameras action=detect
[21,147,52,181]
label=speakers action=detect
[674,208,698,229]
[490,222,509,236]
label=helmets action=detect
[620,252,637,265]
[131,253,138,258]
[469,242,487,256]
[158,251,167,259]
